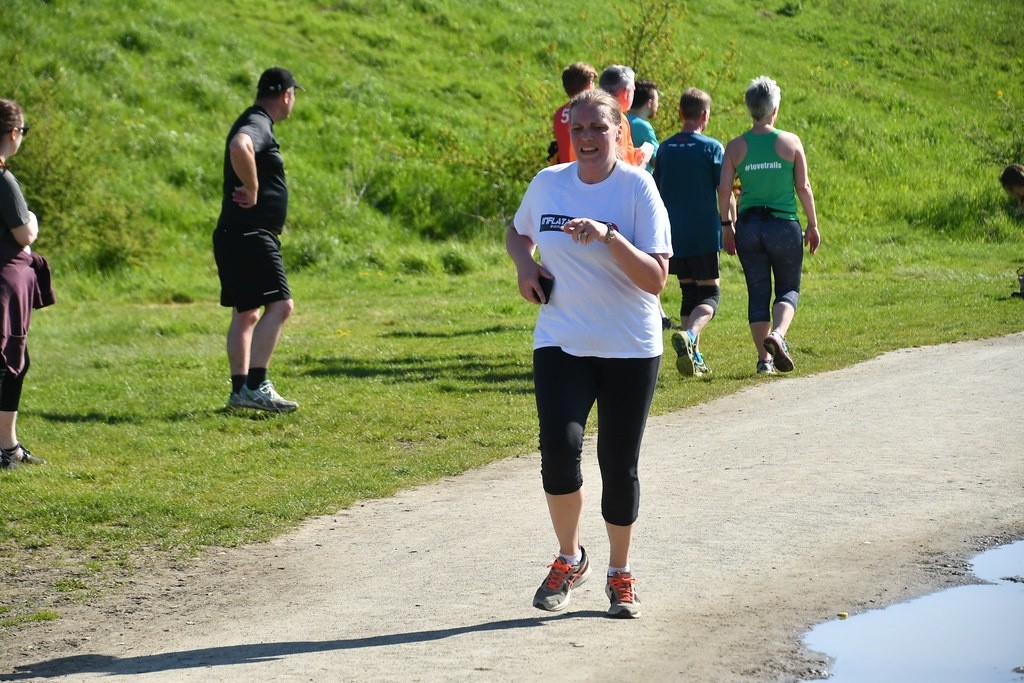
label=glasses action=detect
[16,127,30,136]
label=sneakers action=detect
[763,331,795,373]
[670,331,695,378]
[228,391,239,416]
[239,380,299,412]
[532,546,592,611]
[694,350,712,378]
[756,361,775,375]
[605,572,642,619]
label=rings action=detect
[581,233,587,237]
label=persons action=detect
[0,100,55,465]
[719,77,821,374]
[212,67,305,411]
[553,62,597,163]
[506,87,673,618]
[599,64,645,168]
[657,86,738,376]
[627,79,659,177]
[1000,164,1024,196]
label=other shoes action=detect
[662,317,679,331]
[0,444,47,471]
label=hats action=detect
[257,67,306,95]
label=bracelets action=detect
[604,222,614,243]
[721,221,731,226]
